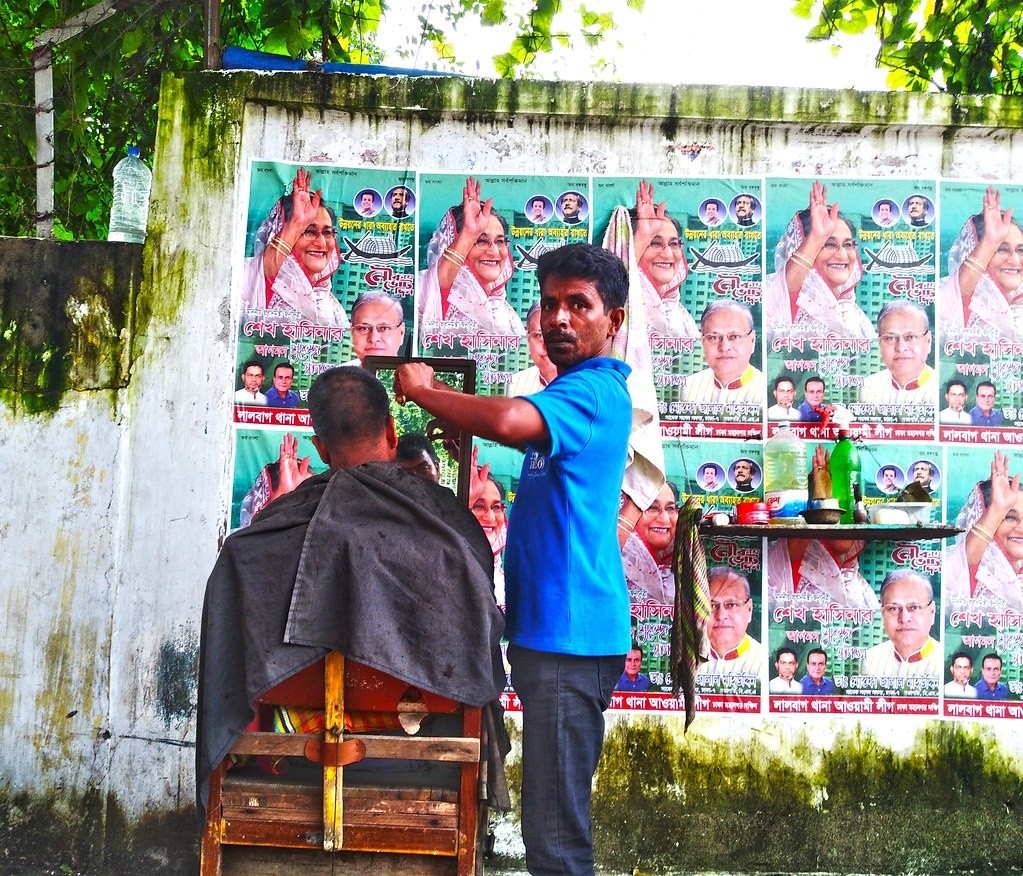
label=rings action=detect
[468,197,474,200]
[815,201,821,204]
[299,188,305,191]
[997,472,1002,475]
[989,206,994,209]
[641,200,646,203]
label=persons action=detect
[943,652,1008,700]
[626,179,700,336]
[612,567,763,691]
[233,431,510,690]
[236,290,1023,426]
[689,461,945,703]
[359,187,930,226]
[942,448,1023,617]
[761,180,878,338]
[937,184,1023,338]
[414,176,529,338]
[614,481,680,605]
[194,246,632,876]
[242,167,351,329]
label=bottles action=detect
[830,430,861,523]
[109,148,152,243]
[763,420,808,525]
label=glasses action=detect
[301,226,338,241]
[471,502,507,515]
[821,239,857,254]
[995,243,1023,257]
[643,503,680,516]
[527,331,543,342]
[1002,511,1023,525]
[352,321,403,335]
[474,235,510,250]
[701,329,753,344]
[648,238,685,252]
[711,598,750,611]
[882,601,932,615]
[878,330,928,344]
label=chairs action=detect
[199,647,480,876]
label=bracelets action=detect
[790,251,814,272]
[963,255,986,275]
[270,237,292,257]
[617,514,635,534]
[442,247,466,268]
[635,256,639,265]
[971,523,994,544]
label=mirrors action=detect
[364,355,475,498]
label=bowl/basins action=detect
[798,508,846,524]
